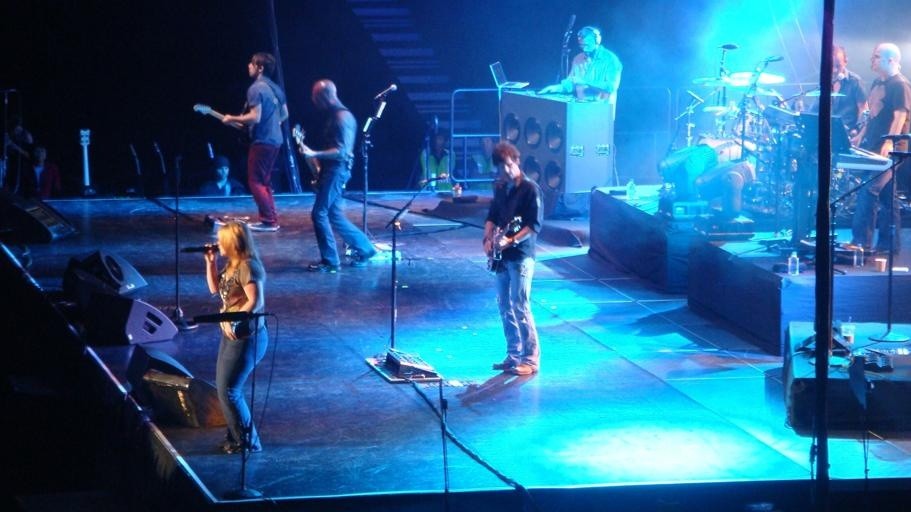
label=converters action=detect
[610,190,626,195]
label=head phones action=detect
[584,26,602,44]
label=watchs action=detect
[512,236,519,247]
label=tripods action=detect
[739,130,848,276]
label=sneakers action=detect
[247,221,276,231]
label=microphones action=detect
[687,89,705,104]
[882,133,911,140]
[567,13,576,30]
[375,83,398,101]
[833,73,845,83]
[180,245,218,253]
[770,55,785,62]
[430,173,447,181]
[721,44,738,50]
[192,311,252,325]
[889,150,911,158]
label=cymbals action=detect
[792,90,846,98]
[725,73,784,86]
[703,105,729,112]
[694,76,735,88]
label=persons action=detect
[483,146,545,375]
[539,25,623,124]
[412,128,456,189]
[849,43,911,255]
[181,220,269,451]
[26,144,62,198]
[804,44,869,145]
[300,80,380,273]
[222,53,290,231]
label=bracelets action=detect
[483,236,492,244]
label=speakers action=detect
[500,89,614,214]
[81,248,149,296]
[125,345,195,386]
[86,290,179,346]
[143,369,232,429]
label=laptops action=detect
[489,61,530,90]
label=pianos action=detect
[763,106,894,171]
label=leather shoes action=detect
[493,360,512,371]
[309,262,342,271]
[350,257,372,268]
[515,364,536,375]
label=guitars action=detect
[194,104,253,146]
[292,124,321,193]
[486,216,523,272]
